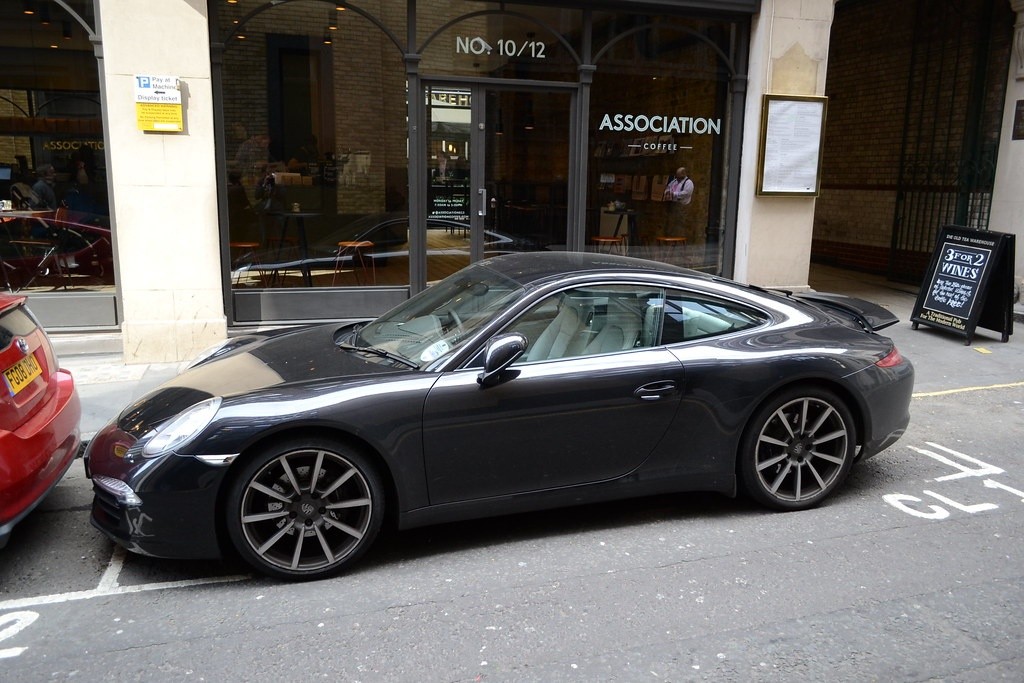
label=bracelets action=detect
[78,165,84,169]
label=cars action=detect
[0,293,83,548]
[0,209,112,287]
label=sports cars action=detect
[82,251,914,585]
[231,213,564,293]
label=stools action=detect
[592,237,623,255]
[230,242,269,288]
[655,237,691,270]
[331,240,377,286]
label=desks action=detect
[267,211,325,289]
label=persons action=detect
[28,164,80,270]
[455,156,470,179]
[252,127,296,173]
[289,135,320,174]
[435,151,452,177]
[69,143,104,226]
[227,117,273,224]
[656,168,694,238]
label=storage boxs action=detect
[291,176,301,185]
[275,172,300,184]
[281,175,291,185]
[303,176,312,185]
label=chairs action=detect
[8,207,75,292]
[526,287,595,362]
[581,295,644,355]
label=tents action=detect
[406,96,484,137]
